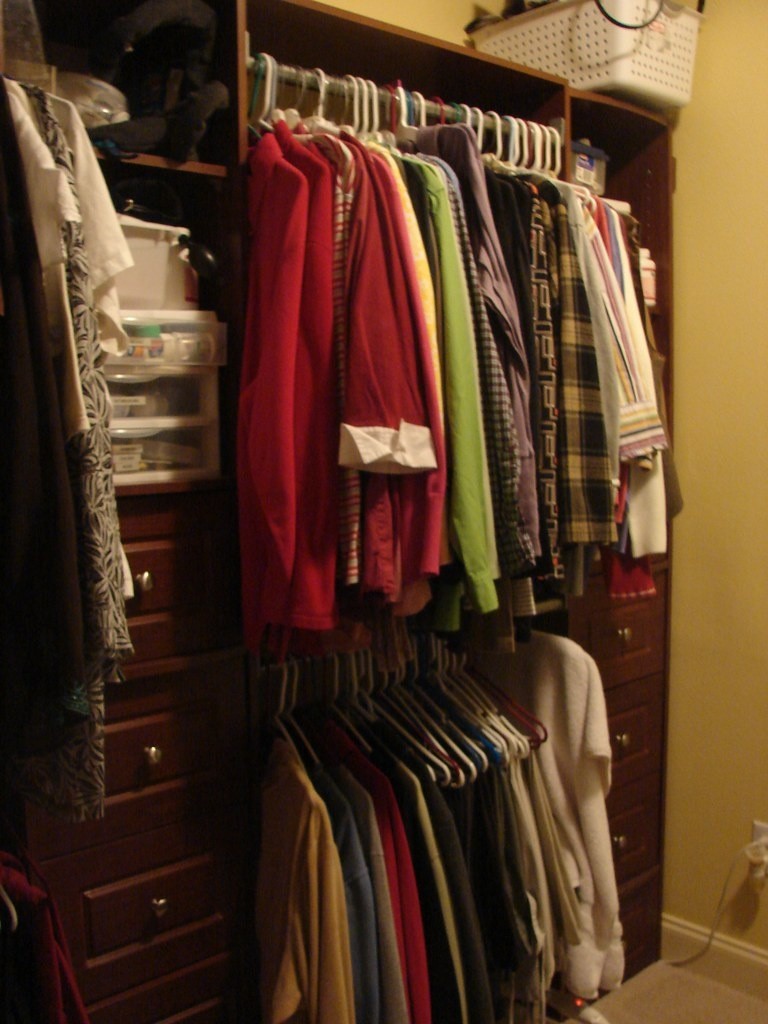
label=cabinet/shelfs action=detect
[0,0,678,1024]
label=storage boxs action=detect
[99,220,229,484]
[466,0,704,110]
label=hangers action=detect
[260,628,549,791]
[244,51,598,211]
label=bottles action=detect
[639,247,657,306]
